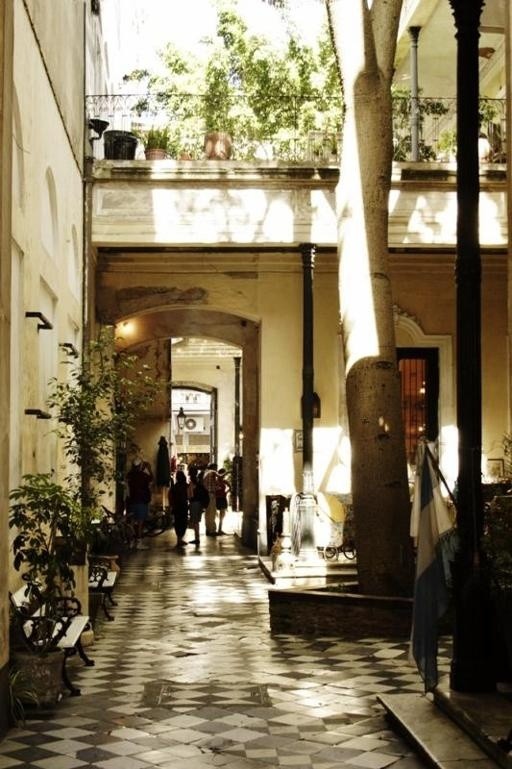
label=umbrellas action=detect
[154,435,171,515]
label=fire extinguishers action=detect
[170,456,177,473]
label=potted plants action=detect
[391,134,437,162]
[177,141,195,161]
[7,467,106,708]
[189,49,234,160]
[41,471,103,567]
[142,131,169,160]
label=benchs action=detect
[9,577,95,697]
[87,558,118,620]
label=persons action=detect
[124,458,235,548]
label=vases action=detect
[103,129,136,160]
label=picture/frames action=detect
[488,458,505,478]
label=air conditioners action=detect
[181,416,204,433]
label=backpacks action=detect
[194,484,210,510]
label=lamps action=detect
[175,406,184,434]
